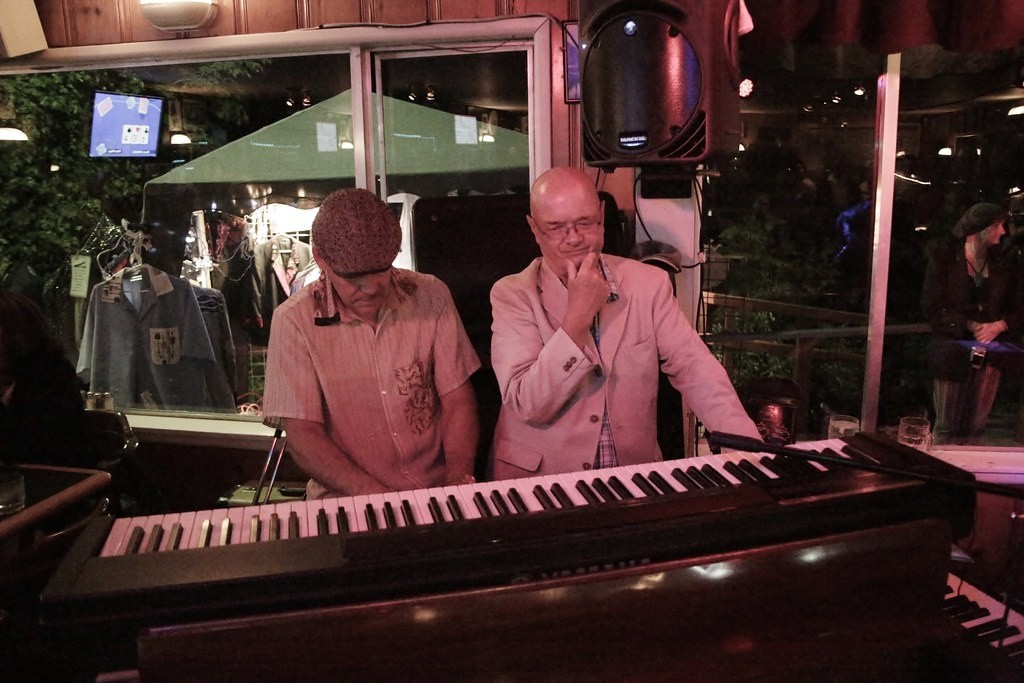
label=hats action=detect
[629,240,682,274]
[312,190,402,276]
[953,204,1007,240]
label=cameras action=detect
[969,345,987,369]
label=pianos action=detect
[42,428,978,683]
[943,568,1024,683]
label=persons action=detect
[261,186,486,504]
[0,289,124,519]
[829,174,1024,446]
[487,163,770,483]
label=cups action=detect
[896,416,933,454]
[0,472,25,516]
[828,414,860,440]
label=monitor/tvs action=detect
[87,89,165,159]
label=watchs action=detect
[443,472,476,485]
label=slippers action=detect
[530,215,602,241]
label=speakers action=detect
[581,0,738,172]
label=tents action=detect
[140,89,529,229]
